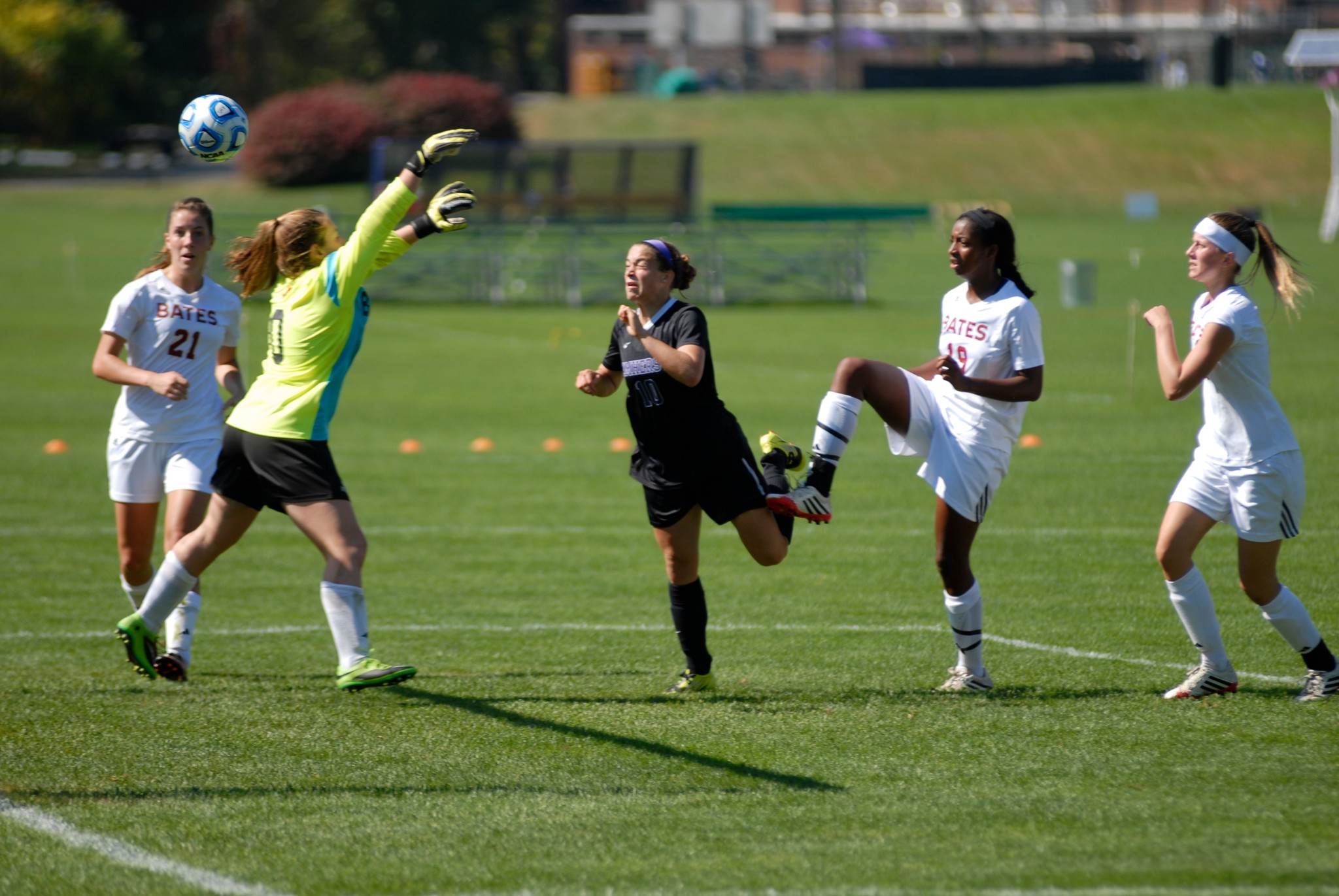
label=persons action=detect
[117,129,476,696]
[1145,212,1339,707]
[576,240,804,695]
[94,196,247,682]
[763,207,1044,692]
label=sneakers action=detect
[336,648,418,693]
[767,471,834,524]
[113,612,159,680]
[1292,658,1339,702]
[137,636,166,676]
[1164,666,1239,699]
[154,652,188,682]
[930,666,993,694]
[758,429,807,472]
[661,670,716,698]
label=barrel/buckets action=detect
[1062,260,1096,304]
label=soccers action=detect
[178,93,250,163]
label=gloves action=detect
[404,128,481,179]
[408,181,479,239]
